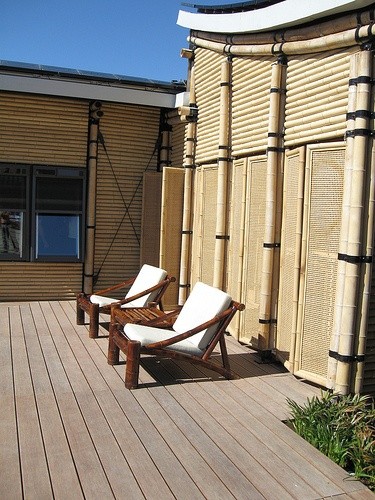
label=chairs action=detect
[103,281,246,392]
[74,263,176,338]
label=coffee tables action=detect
[110,300,175,324]
